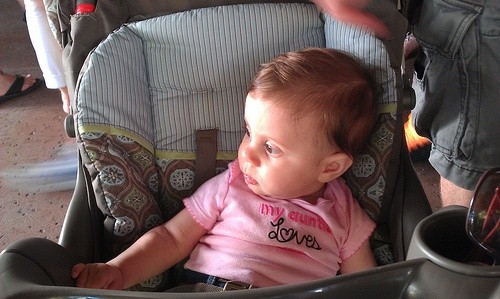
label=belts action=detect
[177,269,249,290]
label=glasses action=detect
[465,166,500,267]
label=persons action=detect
[311,0,500,208]
[21,0,73,117]
[68,43,385,293]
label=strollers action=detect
[0,0,499,299]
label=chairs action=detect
[58,0,409,264]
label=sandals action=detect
[0,70,44,103]
